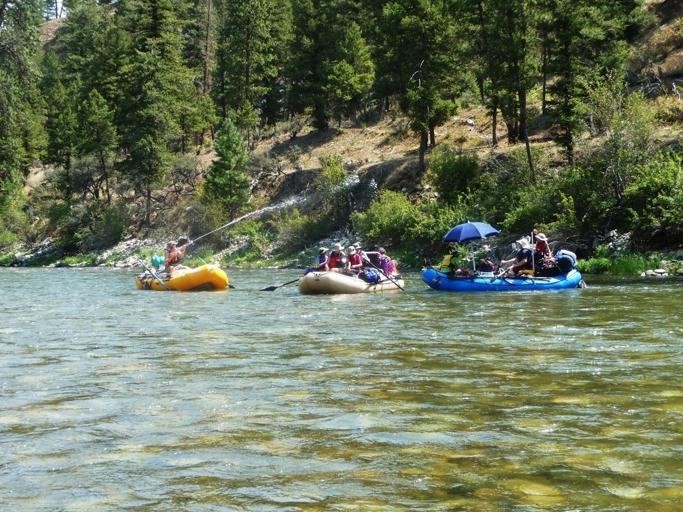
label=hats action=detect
[535,232,546,240]
[516,238,527,247]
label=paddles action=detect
[196,256,234,289]
[259,281,299,292]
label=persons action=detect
[145,255,165,274]
[440,228,552,278]
[315,241,396,278]
[163,240,191,278]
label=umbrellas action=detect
[440,220,501,270]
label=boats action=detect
[136,262,229,291]
[299,257,404,294]
[420,248,582,290]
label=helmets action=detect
[318,241,385,256]
